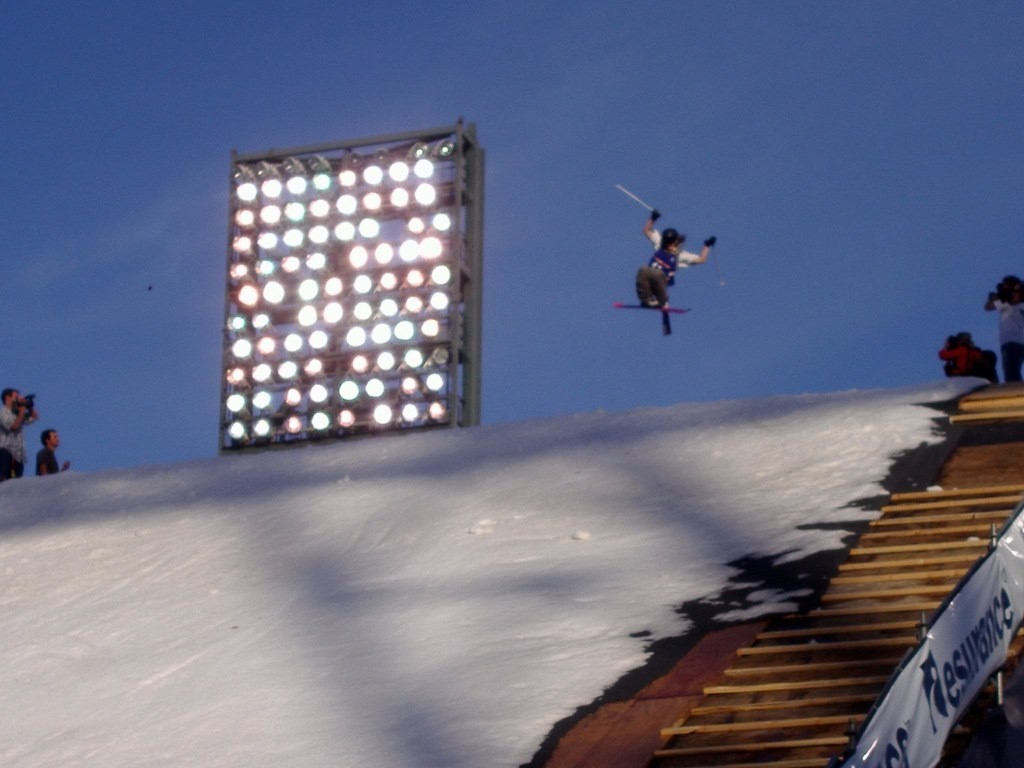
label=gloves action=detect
[705,236,715,246]
[652,210,659,220]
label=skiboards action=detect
[612,298,693,336]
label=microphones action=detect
[23,394,36,400]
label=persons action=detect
[0,387,40,482]
[984,273,1024,386]
[635,210,716,311]
[35,429,70,476]
[938,331,999,383]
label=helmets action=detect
[662,228,678,241]
